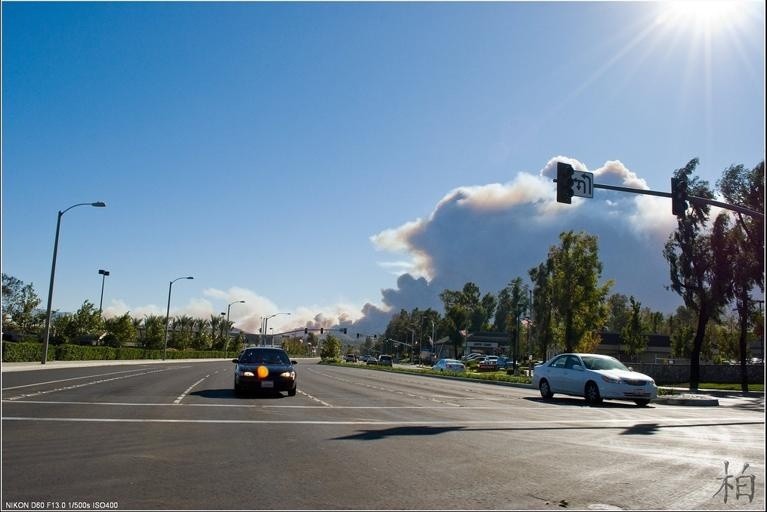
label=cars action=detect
[233,348,298,396]
[432,359,465,372]
[533,353,650,405]
[462,353,515,369]
[346,353,393,367]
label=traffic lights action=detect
[671,177,688,216]
[556,162,574,205]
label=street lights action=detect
[224,300,245,358]
[163,276,193,359]
[40,202,109,364]
[263,315,275,346]
